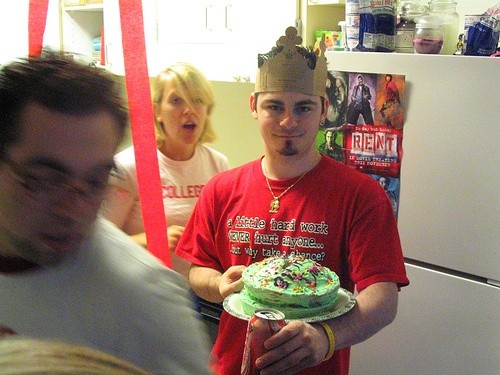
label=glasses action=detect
[0,149,118,209]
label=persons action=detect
[321,72,346,128]
[380,74,400,126]
[347,76,374,126]
[174,25,409,375]
[99,62,232,314]
[0,47,212,375]
[320,129,343,162]
[378,176,397,218]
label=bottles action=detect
[428,0,459,54]
[393,4,428,53]
[413,15,447,54]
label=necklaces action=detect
[264,151,318,213]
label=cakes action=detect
[239,255,340,320]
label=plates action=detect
[223,287,357,325]
[0,339,148,375]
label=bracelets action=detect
[316,321,335,362]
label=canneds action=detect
[241,308,287,375]
